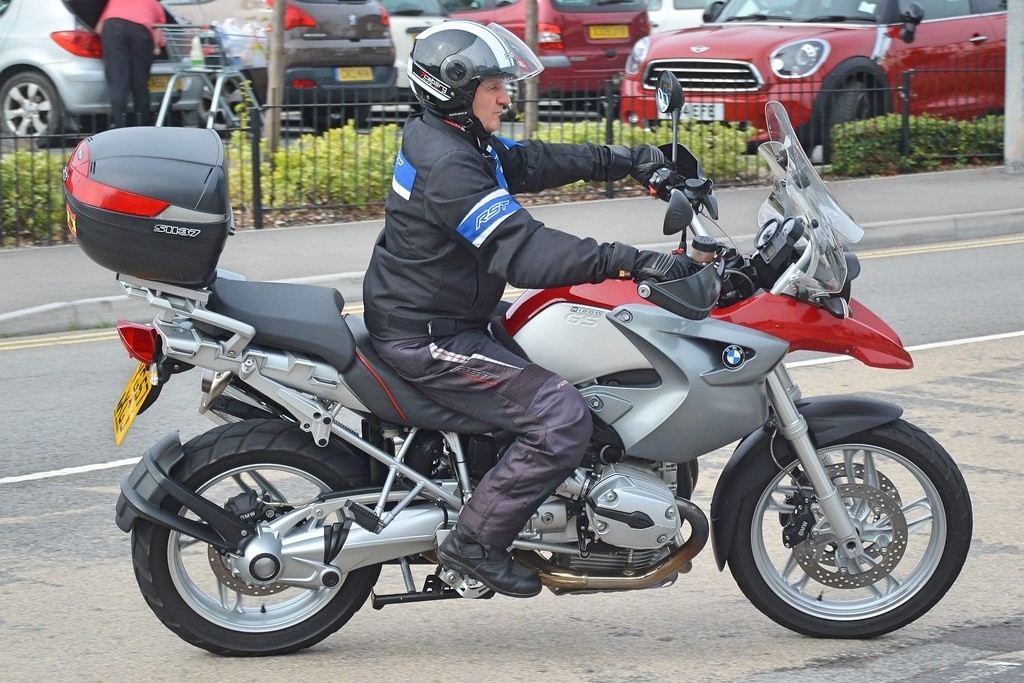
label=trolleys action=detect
[153,18,268,141]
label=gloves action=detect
[608,240,691,281]
[601,144,675,189]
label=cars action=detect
[642,0,763,38]
[621,0,1008,161]
[0,1,187,149]
[451,0,646,120]
[371,0,460,114]
[170,0,396,129]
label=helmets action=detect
[407,19,519,114]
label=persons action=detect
[94,1,166,128]
[362,19,726,598]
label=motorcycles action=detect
[61,70,982,658]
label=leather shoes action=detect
[437,525,542,598]
